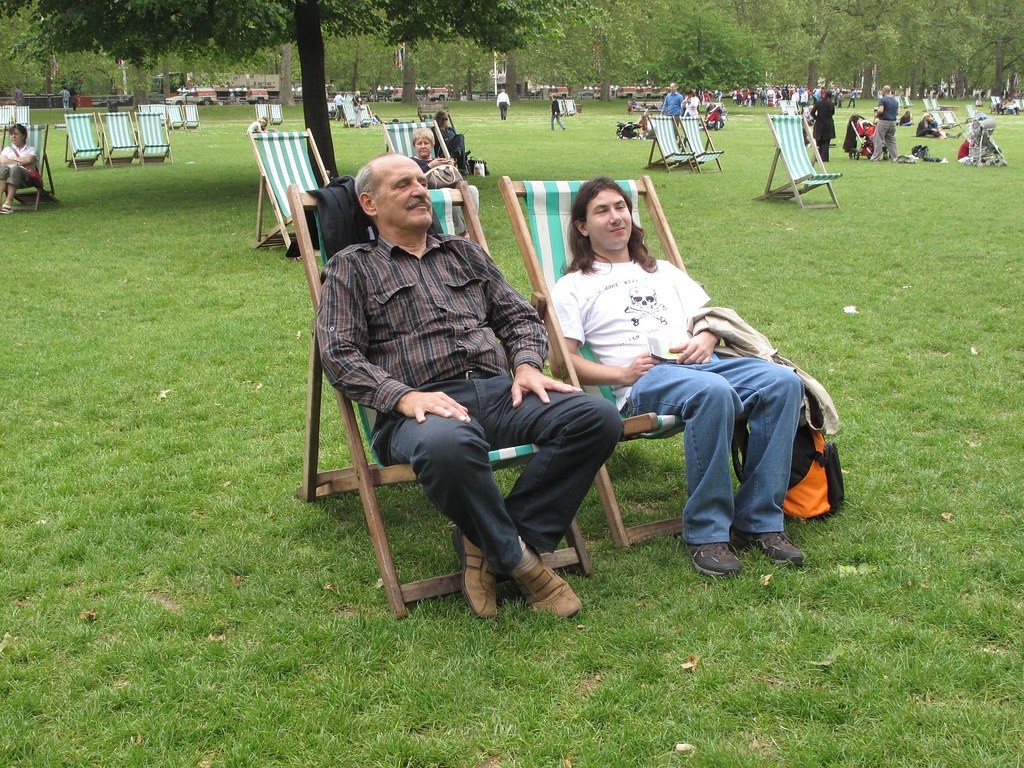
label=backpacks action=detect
[731,416,845,519]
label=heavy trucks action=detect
[147,72,281,104]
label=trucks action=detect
[165,87,269,105]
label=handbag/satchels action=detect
[22,164,43,188]
[425,165,464,190]
[468,156,490,177]
[911,145,930,159]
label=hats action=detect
[922,113,929,119]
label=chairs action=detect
[644,115,695,173]
[678,115,725,172]
[248,127,332,261]
[255,103,284,126]
[754,99,843,211]
[338,92,375,128]
[0,105,59,211]
[418,106,472,176]
[64,104,202,171]
[923,96,1024,131]
[286,181,595,618]
[555,99,579,118]
[894,95,914,107]
[497,174,706,551]
[380,120,451,160]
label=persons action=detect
[550,93,566,131]
[631,83,1024,164]
[14,86,24,106]
[61,86,78,114]
[247,116,268,133]
[551,176,807,577]
[0,127,39,214]
[333,90,479,242]
[497,88,510,120]
[313,152,626,618]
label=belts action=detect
[448,369,501,380]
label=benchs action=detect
[627,100,663,115]
[990,103,1019,114]
[418,101,449,114]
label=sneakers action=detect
[733,526,804,567]
[509,547,582,619]
[452,525,497,619]
[686,543,742,577]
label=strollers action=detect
[848,114,890,160]
[699,105,725,131]
[965,109,1007,168]
[616,107,652,140]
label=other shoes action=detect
[0,204,14,214]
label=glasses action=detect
[441,118,448,121]
